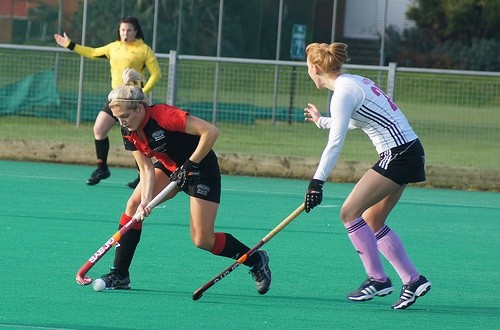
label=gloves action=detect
[305,178,325,213]
[168,159,201,190]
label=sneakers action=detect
[246,249,272,295]
[128,178,140,188]
[390,274,431,310]
[98,266,131,290]
[86,165,110,185]
[348,275,393,301]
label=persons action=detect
[54,16,161,189]
[303,43,431,309]
[98,68,271,295]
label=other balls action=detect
[93,278,106,291]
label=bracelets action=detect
[308,179,325,191]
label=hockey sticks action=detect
[74,178,179,286]
[191,201,305,302]
